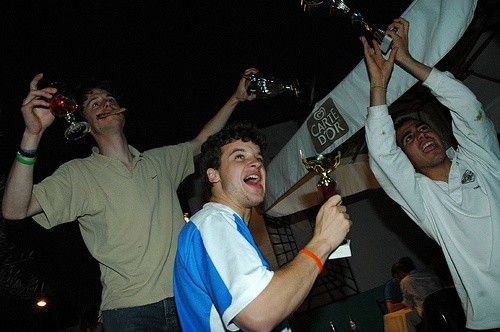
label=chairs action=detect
[376,297,388,314]
[424,285,467,332]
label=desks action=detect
[383,306,421,332]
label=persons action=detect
[359,18,500,332]
[3,67,258,332]
[173,126,353,332]
[384,256,443,321]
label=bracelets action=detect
[298,249,324,274]
[370,85,387,92]
[16,142,39,166]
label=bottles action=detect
[348,314,359,332]
[329,320,338,332]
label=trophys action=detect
[242,68,314,107]
[299,0,401,53]
[304,153,352,259]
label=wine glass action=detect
[244,71,316,105]
[299,143,342,203]
[47,81,92,142]
